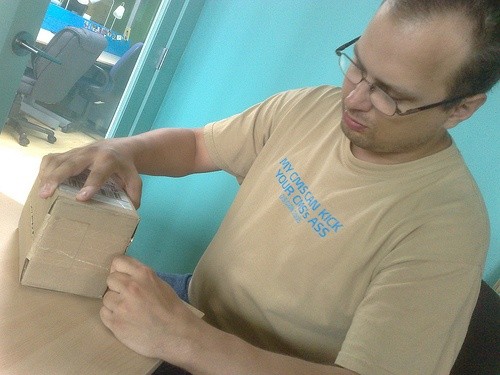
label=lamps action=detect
[108,1,126,33]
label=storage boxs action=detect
[17,153,142,298]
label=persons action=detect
[40,0,499,375]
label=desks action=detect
[0,192,206,374]
[32,23,124,68]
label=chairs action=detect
[62,42,145,140]
[5,26,107,146]
[450,276,500,374]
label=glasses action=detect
[336,29,472,119]
